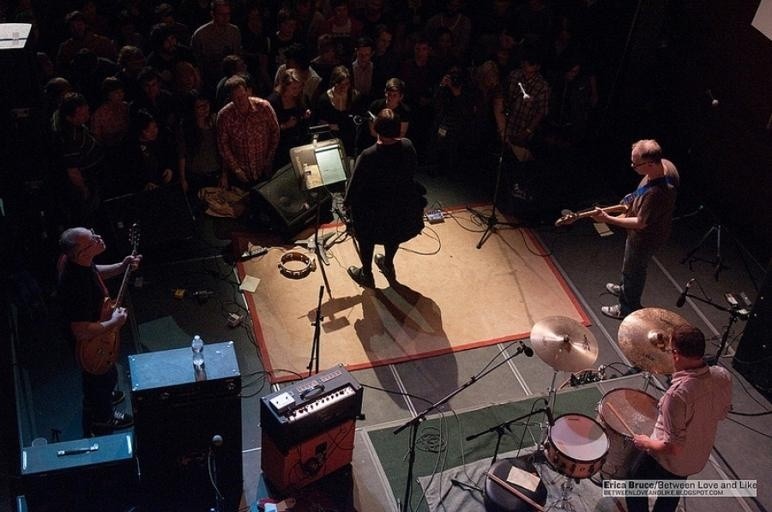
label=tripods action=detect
[306,311,366,420]
[295,190,335,266]
[465,407,545,464]
[680,222,732,282]
[466,96,520,249]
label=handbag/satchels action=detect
[197,170,248,218]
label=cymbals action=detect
[529,315,599,371]
[617,306,689,375]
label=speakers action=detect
[240,162,333,243]
[128,340,244,512]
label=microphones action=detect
[675,278,697,308]
[517,82,532,102]
[541,405,556,427]
[367,111,378,123]
[316,285,324,311]
[706,87,721,107]
[517,340,533,358]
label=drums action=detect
[595,388,660,480]
[544,411,611,479]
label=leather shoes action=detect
[348,265,374,288]
[374,253,396,283]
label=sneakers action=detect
[602,305,626,320]
[606,281,622,297]
[83,392,125,407]
[83,411,134,430]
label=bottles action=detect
[192,335,205,365]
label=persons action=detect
[0,0,213,225]
[624,324,731,512]
[494,0,770,228]
[339,109,418,289]
[214,76,280,190]
[590,139,679,319]
[189,1,525,124]
[55,227,142,370]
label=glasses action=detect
[76,226,98,259]
[631,161,646,170]
[663,346,681,356]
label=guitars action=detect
[76,222,141,376]
[554,193,632,226]
